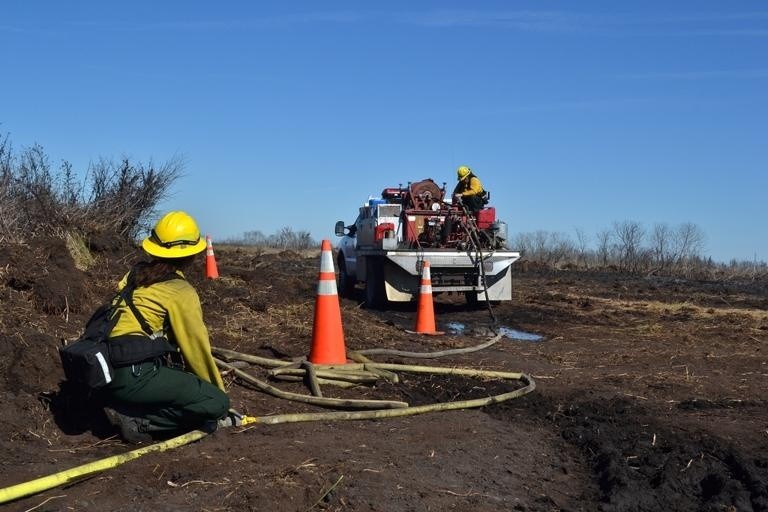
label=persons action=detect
[103,211,230,446]
[451,166,484,213]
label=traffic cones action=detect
[310,239,355,367]
[205,236,219,278]
[404,261,446,336]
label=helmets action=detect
[143,211,207,259]
[458,167,470,181]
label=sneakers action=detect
[105,400,151,442]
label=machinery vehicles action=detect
[336,182,521,309]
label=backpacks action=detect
[59,303,178,390]
